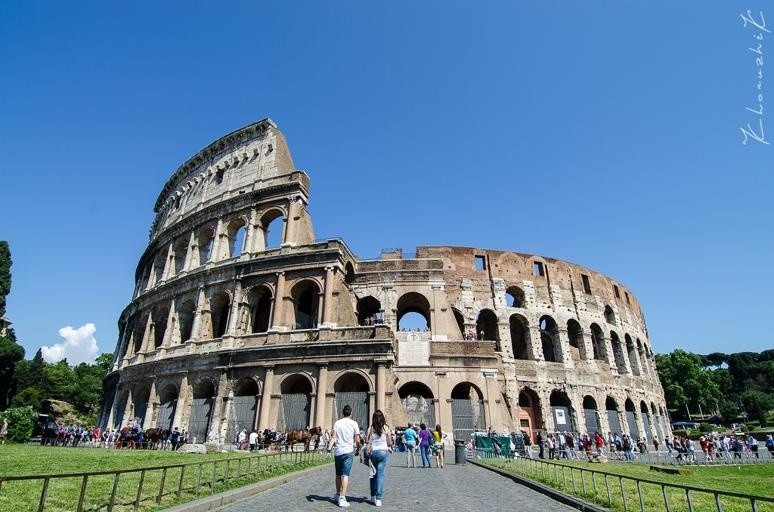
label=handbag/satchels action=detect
[366,443,373,458]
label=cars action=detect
[732,424,742,430]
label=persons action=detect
[432,424,447,469]
[488,427,774,464]
[400,423,418,468]
[417,424,432,468]
[359,410,393,506]
[463,329,485,341]
[327,405,360,506]
[237,426,444,456]
[363,312,383,326]
[32,421,190,452]
[397,327,430,332]
[0,418,11,444]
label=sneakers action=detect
[335,494,350,507]
[369,496,381,507]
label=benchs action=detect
[554,446,773,466]
[233,441,333,451]
[76,439,180,452]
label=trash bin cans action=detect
[453,439,466,466]
[639,443,645,453]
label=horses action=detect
[284,426,323,452]
[146,427,169,451]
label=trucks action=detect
[30,413,58,446]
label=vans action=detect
[669,422,702,432]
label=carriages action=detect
[114,426,171,450]
[240,426,322,454]
